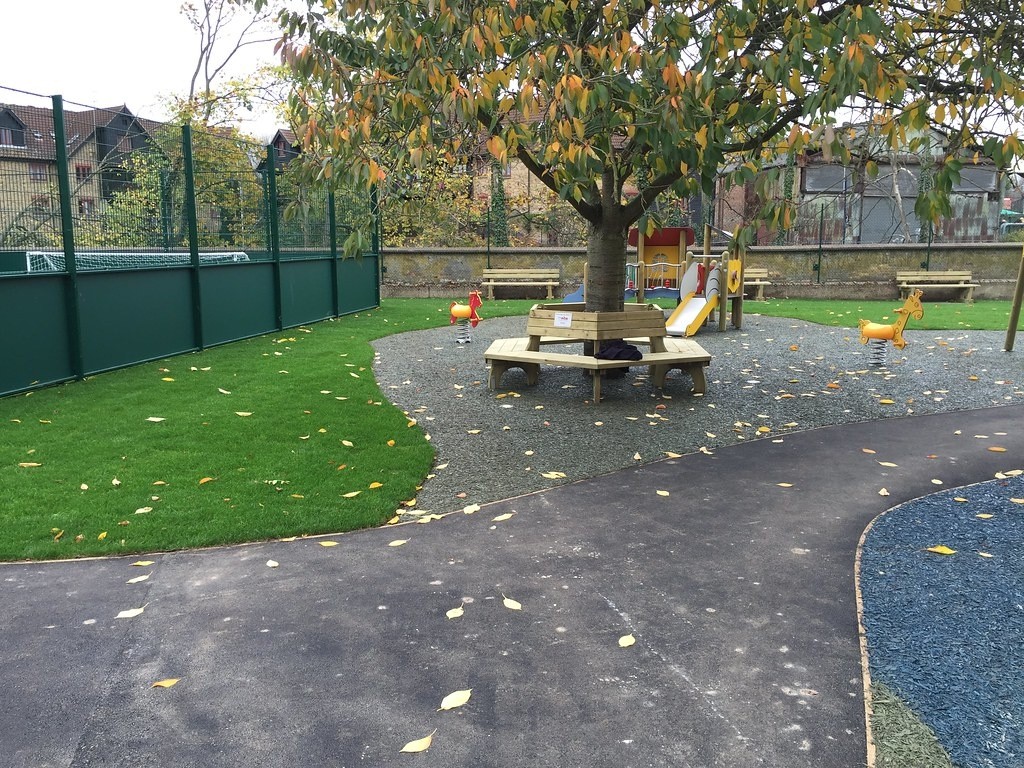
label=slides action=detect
[665,292,720,336]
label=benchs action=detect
[480,269,560,300]
[895,271,982,304]
[744,269,773,302]
[484,335,712,404]
